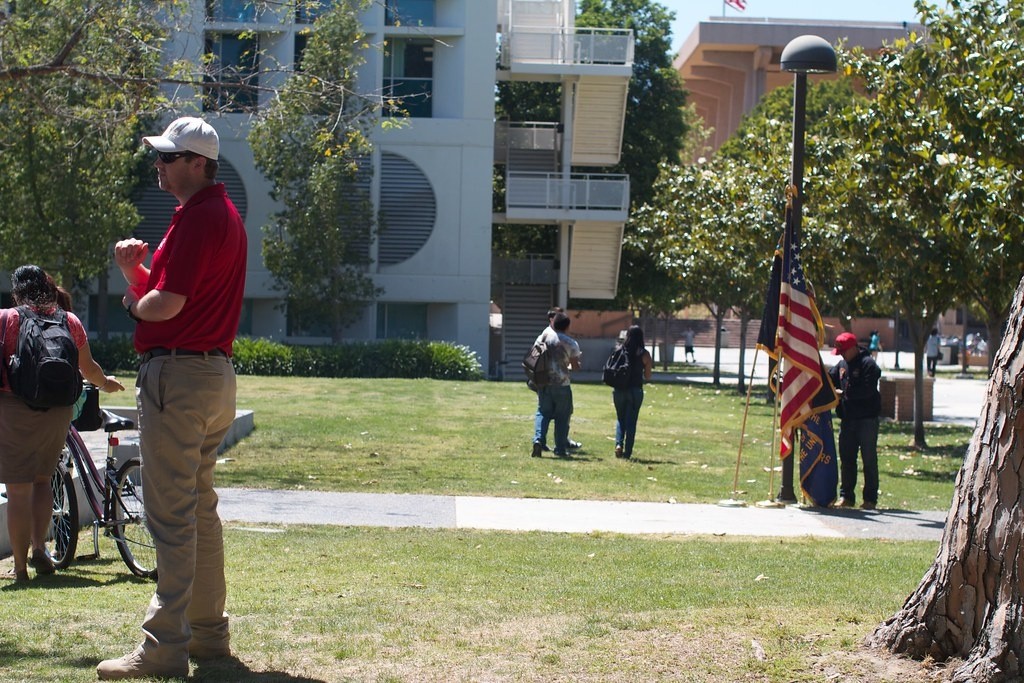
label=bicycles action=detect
[31,377,159,579]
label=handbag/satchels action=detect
[603,348,630,387]
[936,352,943,360]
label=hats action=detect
[142,117,219,160]
[831,332,857,355]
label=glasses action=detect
[157,149,197,163]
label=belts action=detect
[140,347,226,364]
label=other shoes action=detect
[832,498,854,507]
[615,445,622,458]
[566,440,581,448]
[553,451,570,458]
[0,568,29,580]
[532,440,541,457]
[187,639,229,659]
[97,637,189,680]
[32,549,55,574]
[859,501,875,510]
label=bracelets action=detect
[127,302,142,323]
[99,379,109,390]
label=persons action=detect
[826,332,882,510]
[96,118,249,679]
[527,305,582,458]
[681,325,696,363]
[867,328,882,362]
[0,264,125,580]
[925,328,940,378]
[613,325,651,460]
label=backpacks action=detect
[3,305,82,411]
[522,333,552,388]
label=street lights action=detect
[776,36,836,505]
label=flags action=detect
[756,203,839,511]
